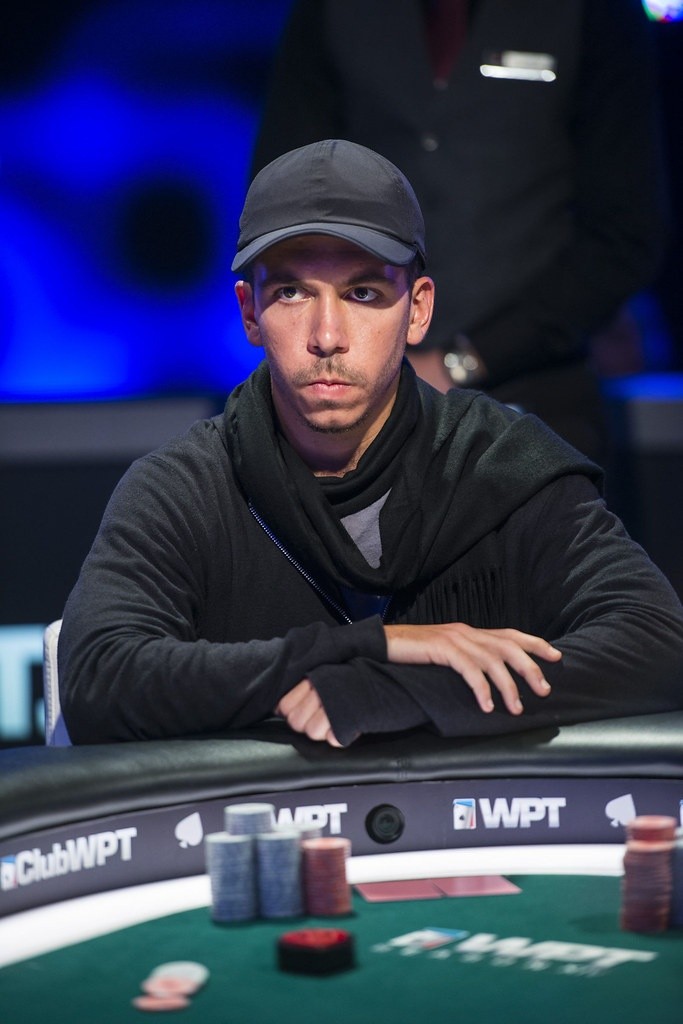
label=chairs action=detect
[43,620,73,746]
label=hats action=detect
[230,139,431,271]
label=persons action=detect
[56,139,683,748]
[249,0,683,505]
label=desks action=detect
[0,870,683,1024]
